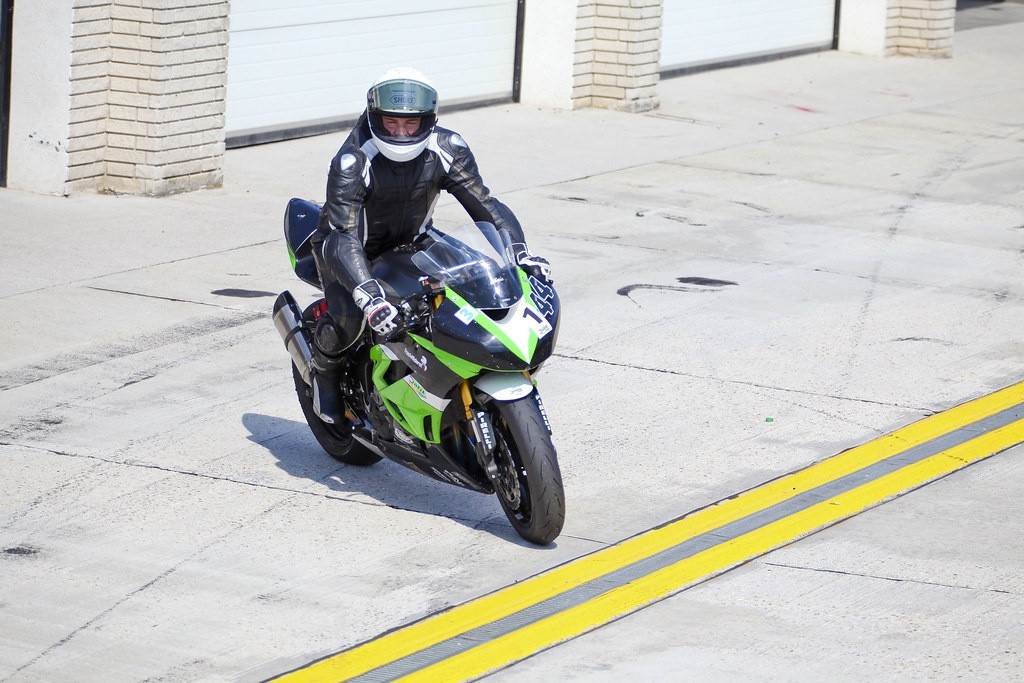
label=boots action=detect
[309,345,345,425]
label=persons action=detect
[308,64,552,425]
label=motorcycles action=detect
[272,196,567,546]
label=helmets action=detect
[366,68,439,163]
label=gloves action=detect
[502,244,551,282]
[352,279,403,337]
